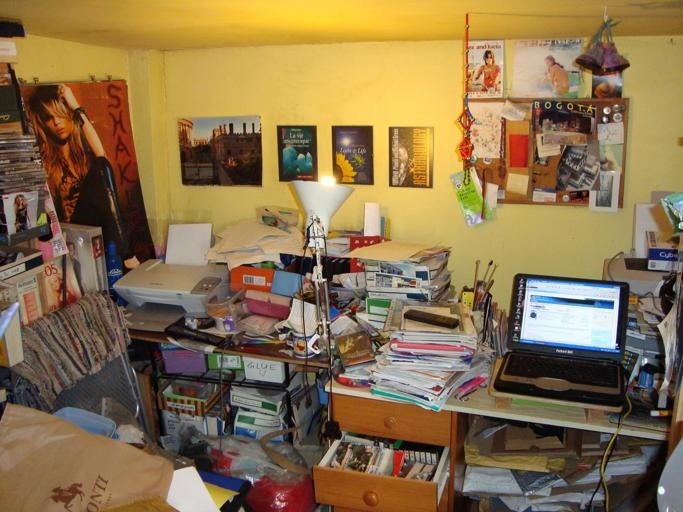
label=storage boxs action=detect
[160,348,290,445]
[644,247,682,274]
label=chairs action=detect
[13,294,155,443]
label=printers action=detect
[111,259,229,334]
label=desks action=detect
[114,325,337,448]
[310,358,682,512]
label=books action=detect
[1,62,109,339]
[370,298,475,413]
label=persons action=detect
[543,55,570,95]
[22,86,140,269]
[474,49,507,93]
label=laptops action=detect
[494,273,630,407]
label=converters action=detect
[325,421,342,440]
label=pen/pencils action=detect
[650,410,672,417]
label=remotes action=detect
[404,310,459,329]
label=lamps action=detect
[291,178,357,512]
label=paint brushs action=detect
[473,260,497,310]
